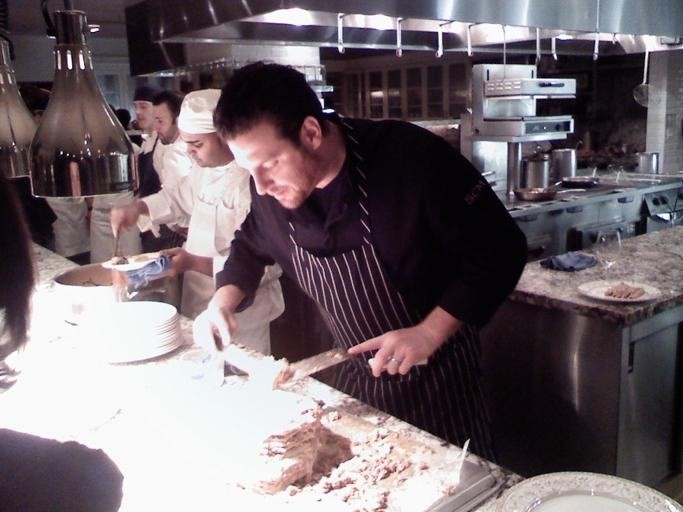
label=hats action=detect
[134,84,162,101]
[178,89,222,135]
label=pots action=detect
[525,146,550,189]
[48,260,168,328]
[551,148,578,179]
[562,174,601,189]
[637,151,659,175]
[512,178,564,204]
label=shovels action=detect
[279,347,427,389]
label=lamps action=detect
[0,1,141,199]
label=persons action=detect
[0,170,124,511]
[192,60,529,469]
[18,86,285,356]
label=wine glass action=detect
[596,230,623,288]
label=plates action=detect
[101,251,162,274]
[84,299,185,366]
[494,470,683,512]
[578,280,661,303]
